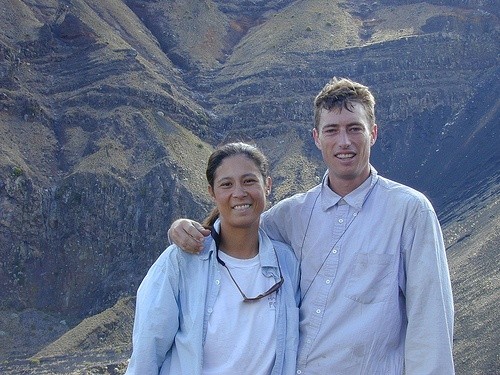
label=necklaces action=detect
[298,177,380,310]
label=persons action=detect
[131,141,302,375]
[165,74,459,374]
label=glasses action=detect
[223,246,284,302]
